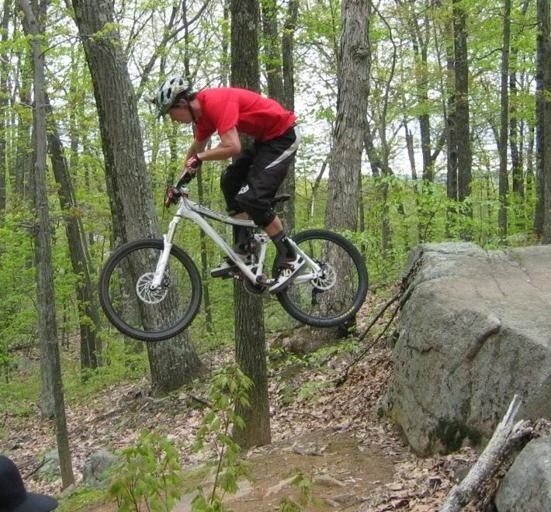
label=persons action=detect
[155,71,307,296]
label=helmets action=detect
[154,77,197,117]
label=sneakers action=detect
[210,253,259,277]
[268,254,305,296]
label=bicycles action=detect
[98,160,369,342]
